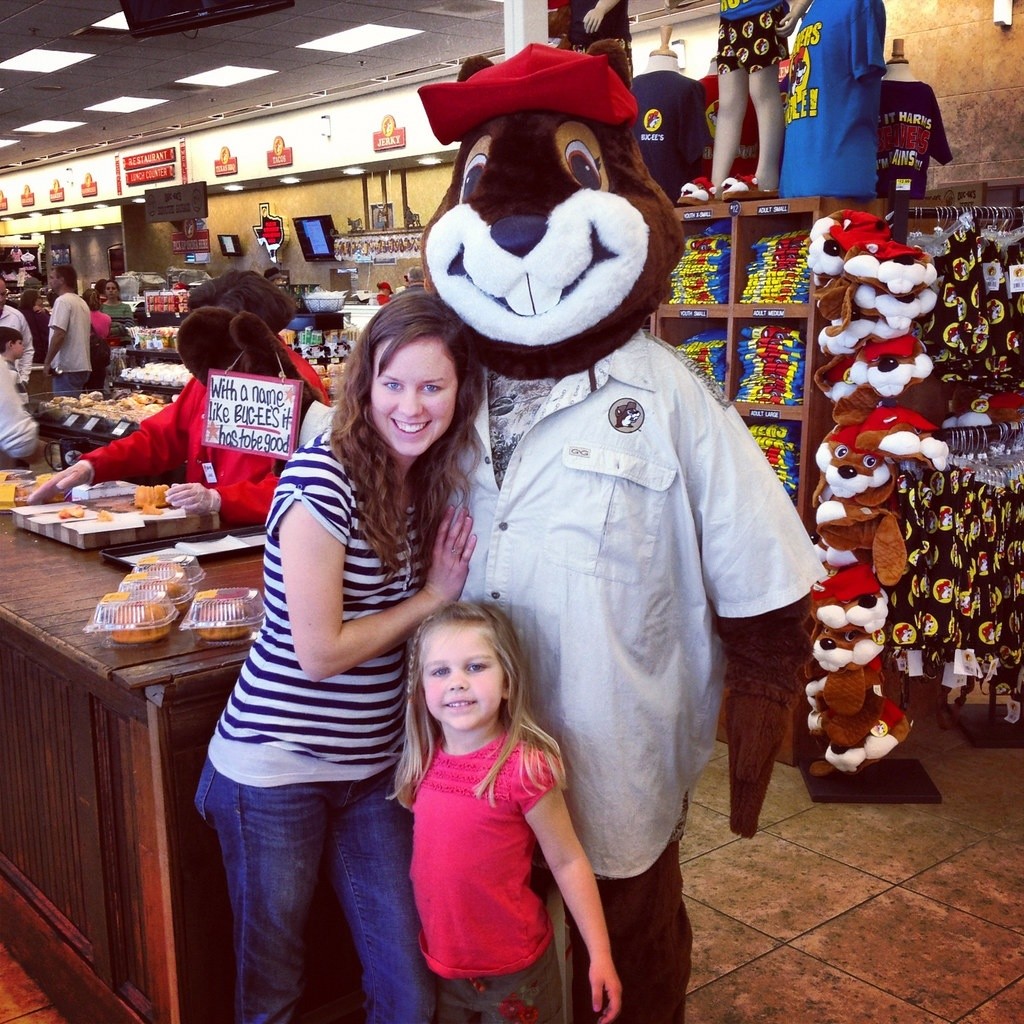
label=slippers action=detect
[721,172,780,202]
[677,176,725,205]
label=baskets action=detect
[303,298,346,313]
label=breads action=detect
[134,484,172,515]
[59,506,85,519]
[108,564,250,644]
[97,509,113,520]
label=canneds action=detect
[301,328,323,344]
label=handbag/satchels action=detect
[89,324,111,369]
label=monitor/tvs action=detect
[51,248,72,265]
[217,235,244,256]
[118,0,295,39]
[292,214,344,262]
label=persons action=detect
[677,0,813,204]
[568,0,633,86]
[776,0,889,200]
[386,601,624,1024]
[18,264,136,398]
[694,59,760,180]
[875,63,954,201]
[0,275,38,472]
[193,285,484,1024]
[26,268,332,526]
[628,56,713,205]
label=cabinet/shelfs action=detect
[643,190,891,535]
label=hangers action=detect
[905,200,1024,486]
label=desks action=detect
[0,465,363,1024]
[110,348,187,396]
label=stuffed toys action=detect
[176,39,829,1024]
[805,211,948,771]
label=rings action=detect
[451,548,457,554]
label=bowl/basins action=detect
[130,555,206,591]
[118,570,198,624]
[178,587,266,647]
[83,591,180,651]
